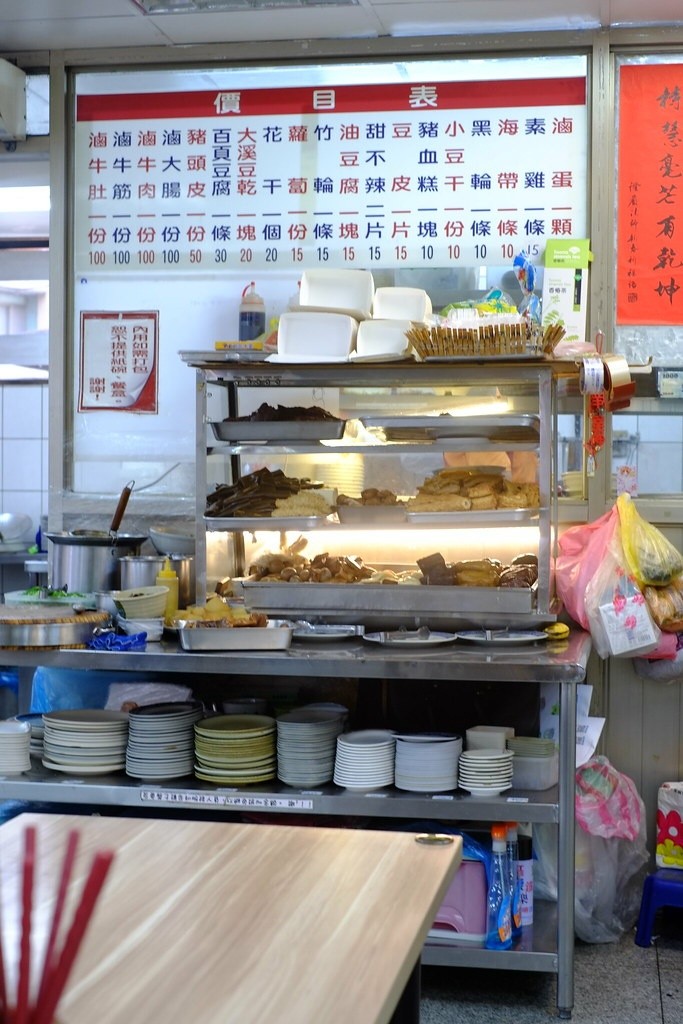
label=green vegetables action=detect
[21,583,83,606]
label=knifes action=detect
[73,602,98,614]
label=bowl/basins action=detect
[225,697,268,716]
[149,525,195,556]
[112,585,169,620]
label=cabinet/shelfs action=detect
[0,628,593,1022]
[189,358,616,629]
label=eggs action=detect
[251,559,311,584]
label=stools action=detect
[634,868,683,948]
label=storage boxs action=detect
[428,860,487,941]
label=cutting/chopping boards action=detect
[0,603,111,652]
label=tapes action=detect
[580,354,631,401]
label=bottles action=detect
[239,281,266,345]
[155,558,179,628]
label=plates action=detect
[292,624,364,640]
[0,702,558,801]
[455,628,549,645]
[363,630,458,648]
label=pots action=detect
[116,554,194,612]
[42,529,149,596]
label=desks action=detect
[1,810,464,1024]
